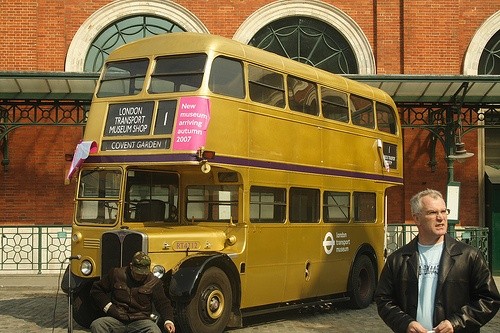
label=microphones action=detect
[68,255,82,260]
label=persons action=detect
[375,189,500,333]
[90,252,175,333]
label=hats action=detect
[131,251,151,275]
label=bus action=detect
[61,32,404,333]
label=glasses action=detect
[420,209,451,216]
